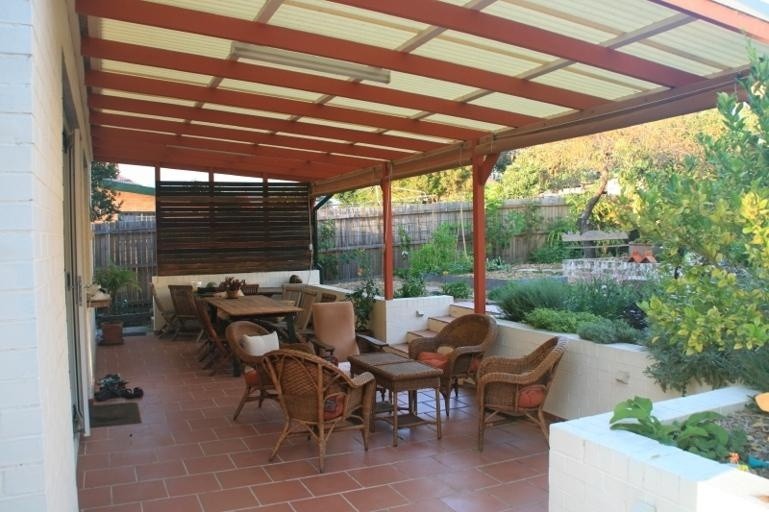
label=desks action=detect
[346,351,444,447]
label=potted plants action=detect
[93,262,143,345]
[343,280,380,349]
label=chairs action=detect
[408,313,497,416]
[310,302,381,379]
[145,280,337,377]
[225,320,313,420]
[475,335,569,452]
[261,348,376,474]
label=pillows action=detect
[519,386,544,408]
[241,330,283,374]
[437,344,478,372]
[418,351,446,369]
[338,360,353,381]
